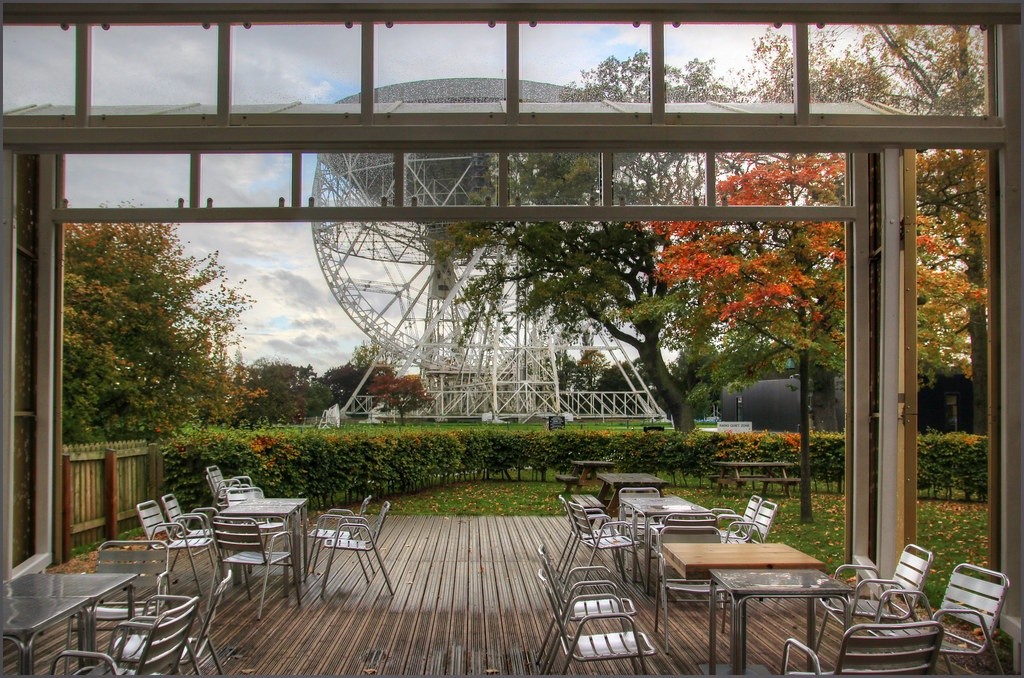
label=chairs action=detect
[537,567,658,675]
[712,494,777,543]
[532,543,638,675]
[43,466,301,675]
[320,501,394,598]
[781,544,1010,675]
[303,495,374,583]
[556,487,660,592]
[654,526,730,654]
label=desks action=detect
[711,461,795,497]
[620,497,711,592]
[220,497,308,597]
[566,461,616,493]
[3,574,138,675]
[662,543,824,675]
[709,568,853,675]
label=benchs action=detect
[707,475,802,482]
[570,495,606,514]
[598,473,669,528]
[556,475,579,483]
